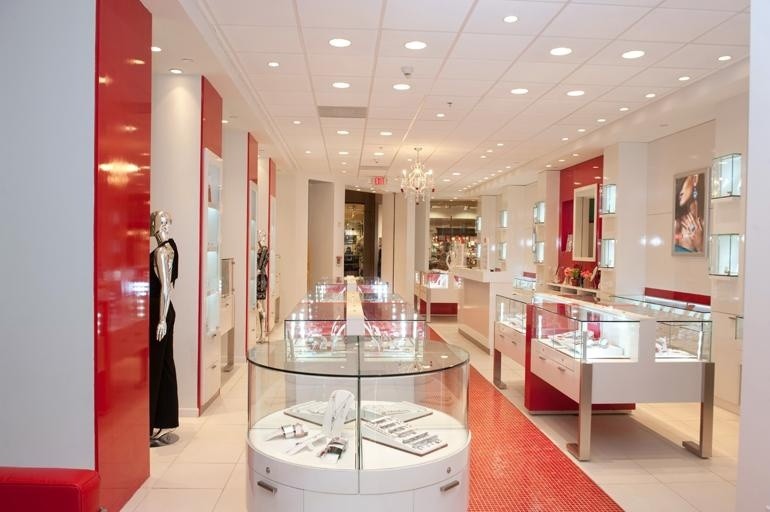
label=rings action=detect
[690,225,696,231]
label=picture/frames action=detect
[672,166,708,258]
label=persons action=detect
[674,172,705,254]
[344,236,364,276]
[432,234,463,269]
[378,237,382,279]
[150,210,179,440]
[255,228,268,344]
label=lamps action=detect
[400,146,435,205]
